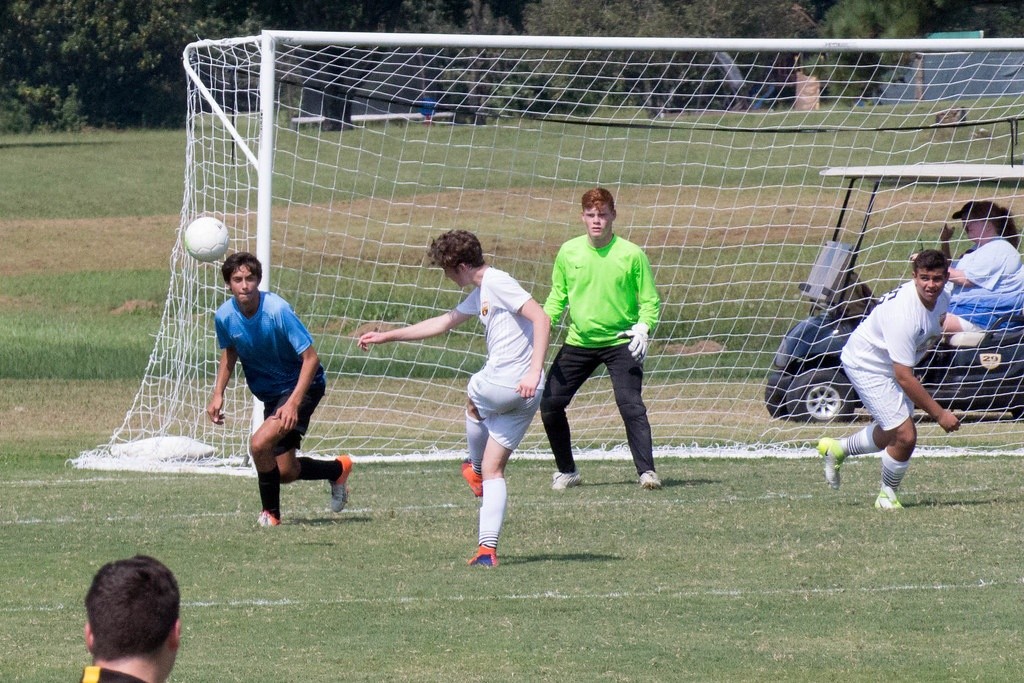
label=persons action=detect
[816,249,962,513]
[207,252,353,530]
[544,189,662,494]
[80,555,182,683]
[356,230,550,566]
[908,201,1024,350]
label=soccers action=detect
[182,215,232,264]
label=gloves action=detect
[617,323,650,364]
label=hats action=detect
[952,201,977,220]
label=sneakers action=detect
[552,470,582,490]
[875,483,905,511]
[328,454,352,513]
[816,437,846,490]
[256,510,280,528]
[640,470,661,489]
[467,545,498,567]
[462,461,483,498]
